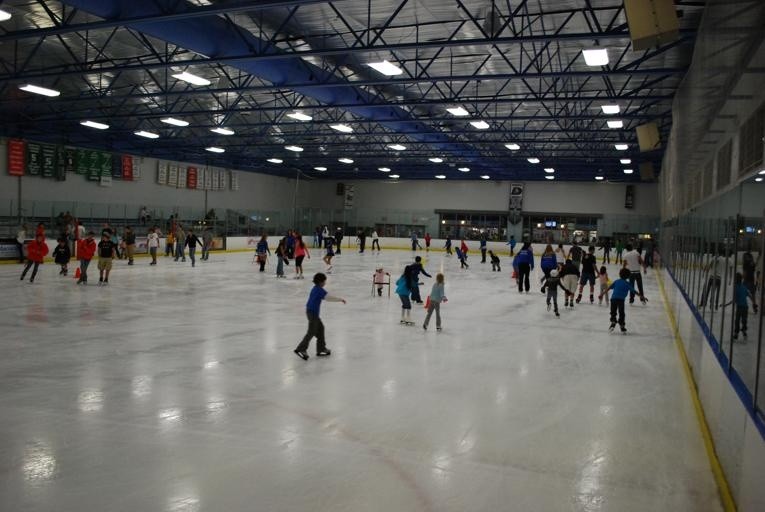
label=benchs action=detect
[0,212,282,259]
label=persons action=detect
[699,245,762,340]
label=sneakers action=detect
[620,326,627,332]
[295,347,309,358]
[316,347,331,353]
[400,318,415,323]
[695,298,759,344]
[608,322,616,329]
[640,298,646,304]
[629,298,634,303]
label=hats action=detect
[102,231,111,239]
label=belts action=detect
[630,271,640,274]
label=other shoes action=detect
[258,266,333,279]
[460,260,609,318]
[21,255,209,285]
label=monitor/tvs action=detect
[545,220,557,228]
[745,226,753,232]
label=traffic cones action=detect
[71,266,82,279]
[422,295,430,309]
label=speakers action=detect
[625,185,633,208]
[337,183,343,195]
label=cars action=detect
[466,226,597,243]
